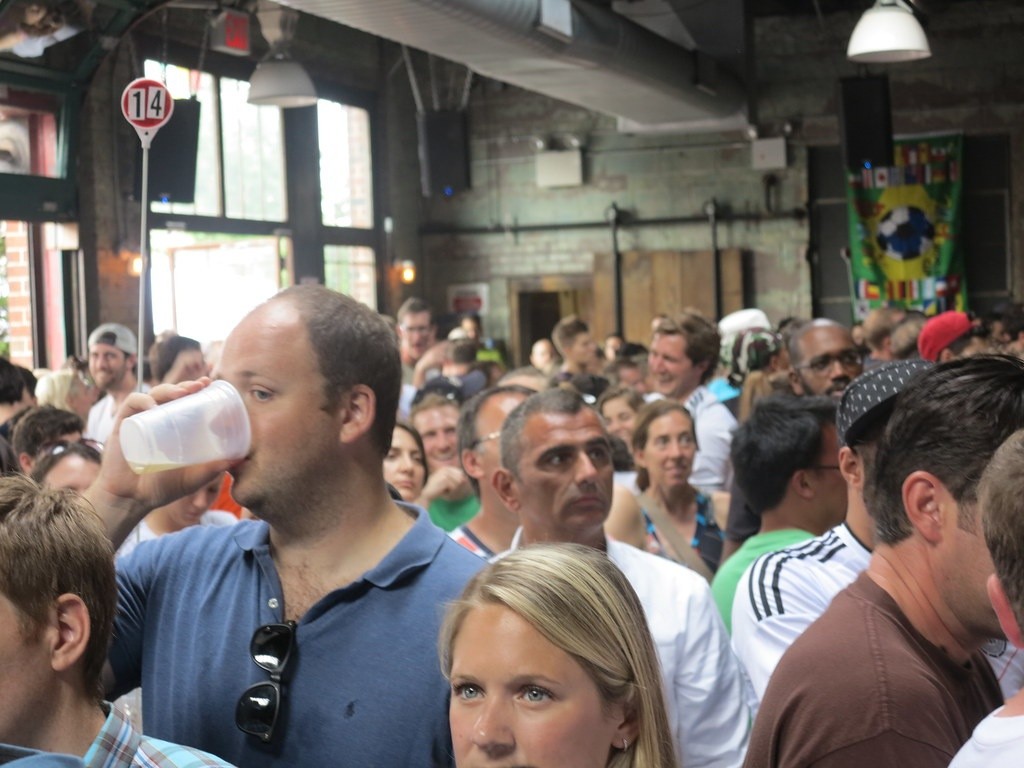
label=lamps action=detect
[743,118,798,172]
[526,127,589,189]
[847,0,932,64]
[248,9,317,110]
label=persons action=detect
[69,282,496,768]
[0,470,239,767]
[494,388,761,768]
[379,294,653,557]
[0,322,264,733]
[439,542,684,767]
[635,308,1024,768]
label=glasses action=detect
[237,622,299,742]
[41,438,102,465]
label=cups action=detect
[119,379,252,476]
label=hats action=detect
[87,323,136,359]
[731,326,779,384]
[920,312,982,364]
[836,358,934,446]
[716,309,771,356]
